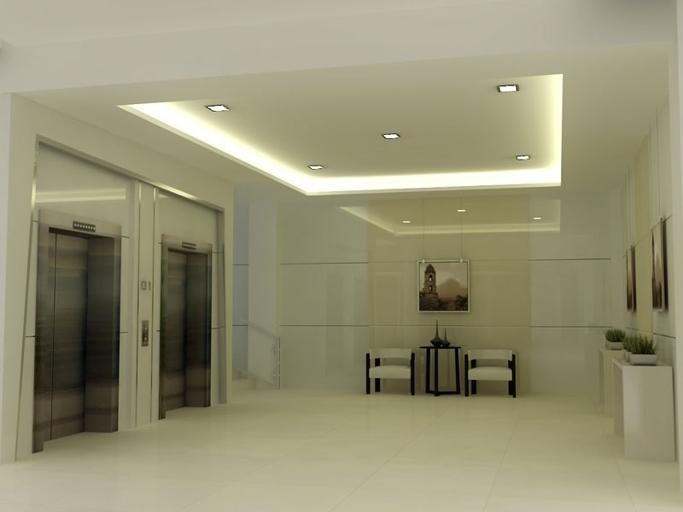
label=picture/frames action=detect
[626,217,665,313]
[416,259,470,313]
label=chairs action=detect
[464,349,517,397]
[366,347,415,395]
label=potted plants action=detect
[605,328,658,365]
